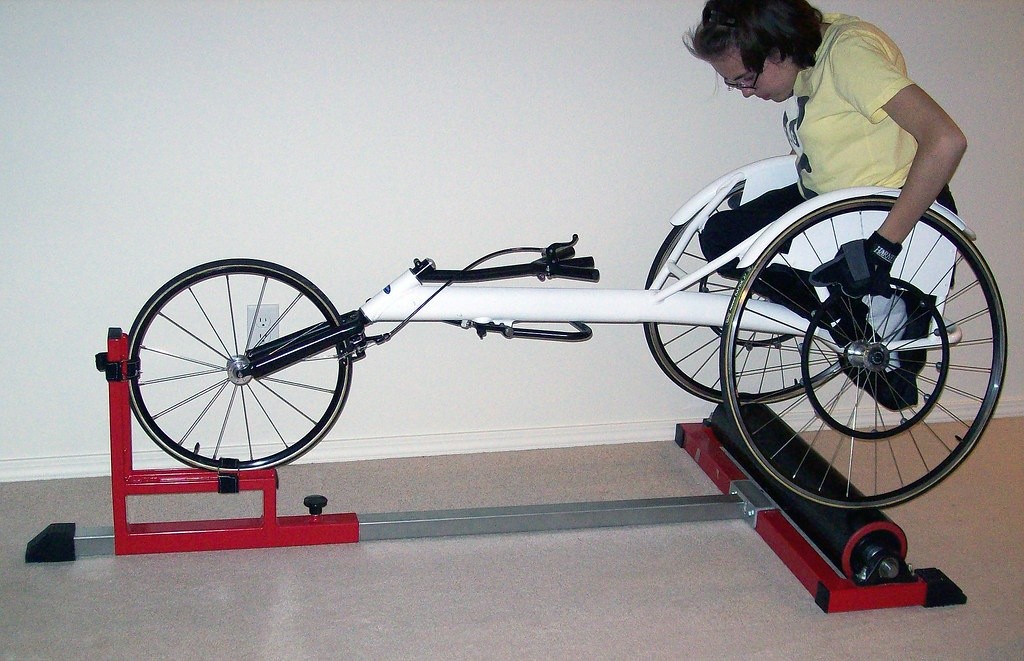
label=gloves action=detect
[809,232,902,300]
[727,190,743,210]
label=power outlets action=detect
[247,304,279,350]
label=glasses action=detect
[724,72,760,93]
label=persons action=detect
[681,0,967,411]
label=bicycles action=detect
[124,153,1008,517]
